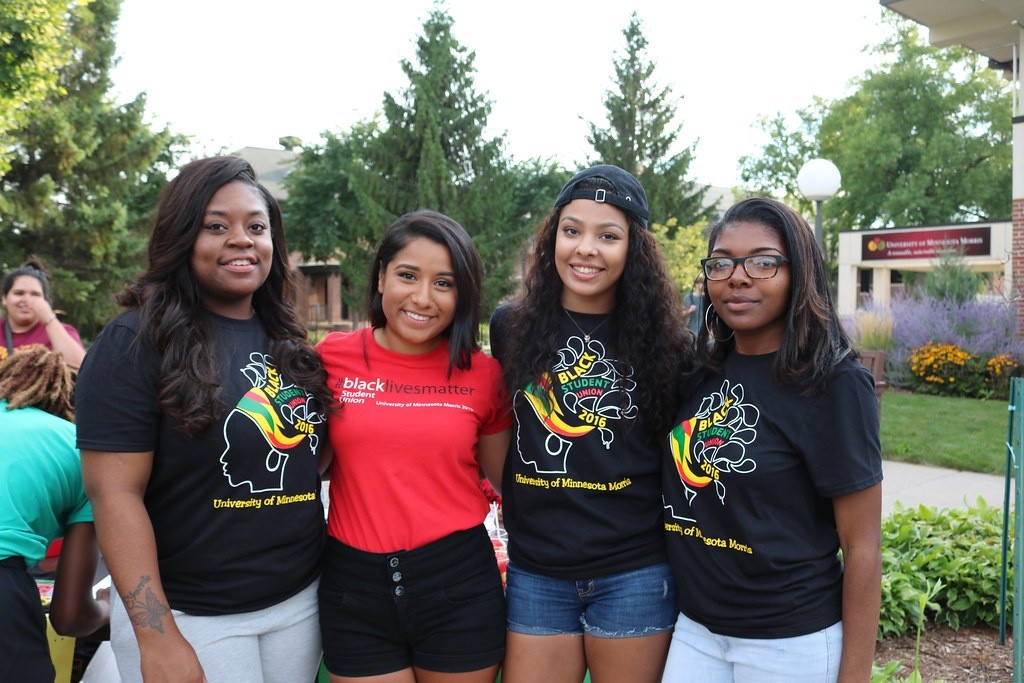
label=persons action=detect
[312,207,513,683]
[488,162,700,683]
[0,265,114,682]
[71,154,330,683]
[662,195,888,683]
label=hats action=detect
[553,164,650,223]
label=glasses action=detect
[703,253,790,281]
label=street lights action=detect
[797,159,841,251]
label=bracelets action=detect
[43,313,55,329]
